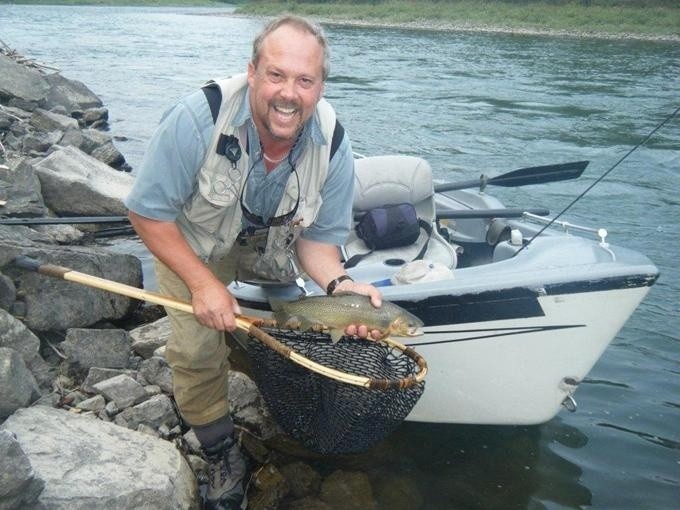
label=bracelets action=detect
[327,275,354,296]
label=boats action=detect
[228,151,661,425]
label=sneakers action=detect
[201,428,253,509]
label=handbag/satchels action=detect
[355,203,420,250]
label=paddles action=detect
[90,161,589,238]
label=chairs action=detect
[341,155,457,276]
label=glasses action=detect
[240,161,301,226]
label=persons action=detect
[125,16,381,510]
